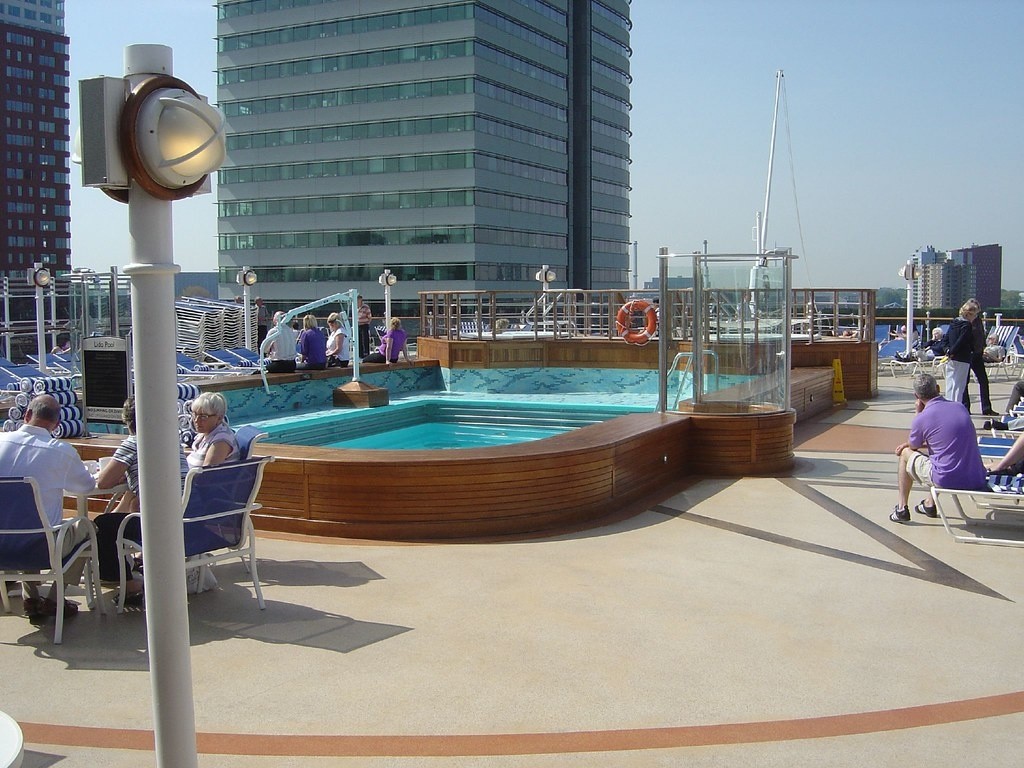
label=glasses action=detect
[192,414,217,420]
[328,321,334,324]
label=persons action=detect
[736,291,751,334]
[186,392,241,471]
[94,394,190,606]
[917,334,1005,363]
[296,315,328,370]
[51,338,70,354]
[251,311,296,375]
[255,297,272,357]
[0,395,99,619]
[984,417,1024,431]
[326,312,349,368]
[843,324,853,336]
[962,298,999,416]
[890,374,987,523]
[652,298,659,337]
[896,327,943,362]
[1006,381,1024,413]
[234,295,244,304]
[991,430,1024,471]
[362,317,414,369]
[357,295,372,358]
[889,325,918,340]
[940,303,978,403]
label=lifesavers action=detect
[616,300,656,343]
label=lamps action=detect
[33,268,51,287]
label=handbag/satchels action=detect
[186,553,217,593]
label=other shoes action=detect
[982,409,999,416]
[24,596,78,617]
[112,590,142,606]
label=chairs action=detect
[455,320,533,334]
[0,295,387,388]
[0,425,276,645]
[827,323,1024,549]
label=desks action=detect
[62,462,129,610]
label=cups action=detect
[99,457,112,471]
[82,460,97,475]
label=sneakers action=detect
[915,500,936,518]
[890,505,911,522]
